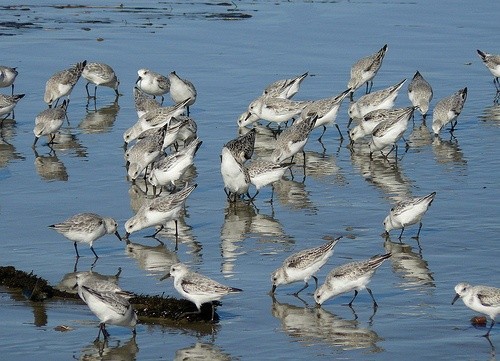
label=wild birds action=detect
[71,271,139,342]
[367,106,416,168]
[28,95,72,150]
[476,48,500,86]
[218,71,352,208]
[42,59,88,109]
[429,85,469,138]
[121,95,191,152]
[81,61,121,110]
[383,190,437,240]
[145,135,204,195]
[166,70,198,114]
[348,107,409,149]
[135,66,170,104]
[348,44,389,102]
[313,251,393,308]
[270,235,344,294]
[169,115,198,150]
[449,281,500,338]
[347,77,408,128]
[124,183,198,239]
[407,69,434,122]
[131,83,163,119]
[46,212,122,259]
[129,183,191,217]
[124,122,168,187]
[159,262,246,320]
[0,90,27,125]
[0,63,20,96]
